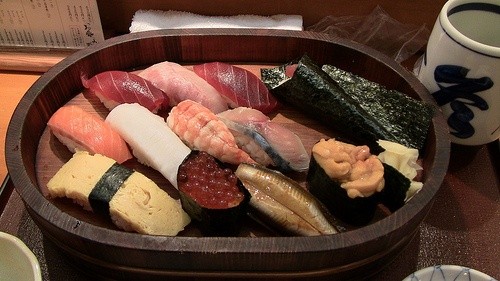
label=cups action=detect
[1,230,42,281]
[419,0,500,145]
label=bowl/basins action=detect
[402,265,498,281]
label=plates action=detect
[5,29,449,281]
